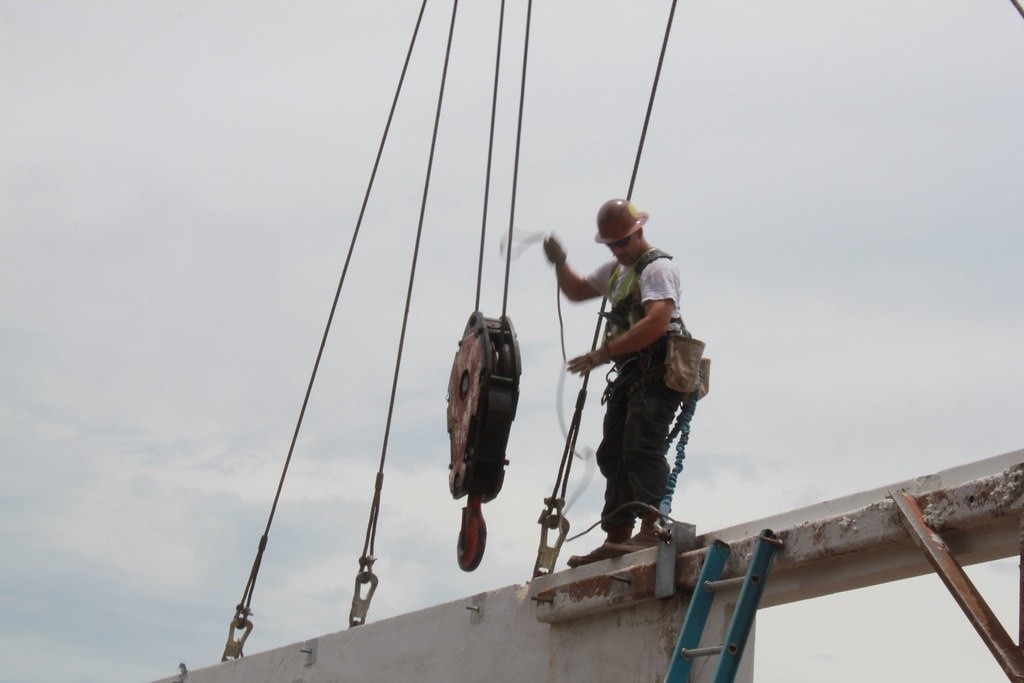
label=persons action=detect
[542,197,681,568]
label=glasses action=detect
[606,231,639,247]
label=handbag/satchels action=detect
[683,358,710,403]
[664,334,705,393]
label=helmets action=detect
[594,200,649,243]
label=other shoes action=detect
[567,525,635,567]
[605,515,661,552]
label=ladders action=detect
[661,528,780,683]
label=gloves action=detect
[567,343,611,377]
[544,237,566,266]
